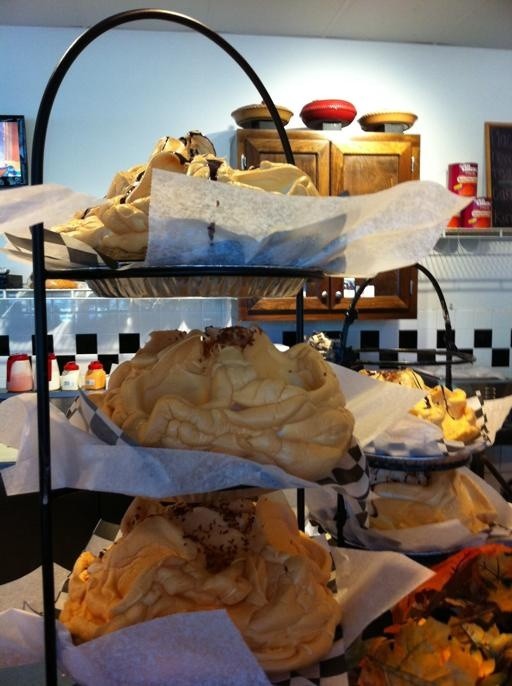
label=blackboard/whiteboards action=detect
[485,121,512,227]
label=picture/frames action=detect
[0,114,29,189]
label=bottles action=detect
[85,360,106,390]
[61,362,82,391]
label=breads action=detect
[51,131,512,686]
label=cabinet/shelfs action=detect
[236,128,421,321]
[334,263,511,570]
[30,8,328,686]
